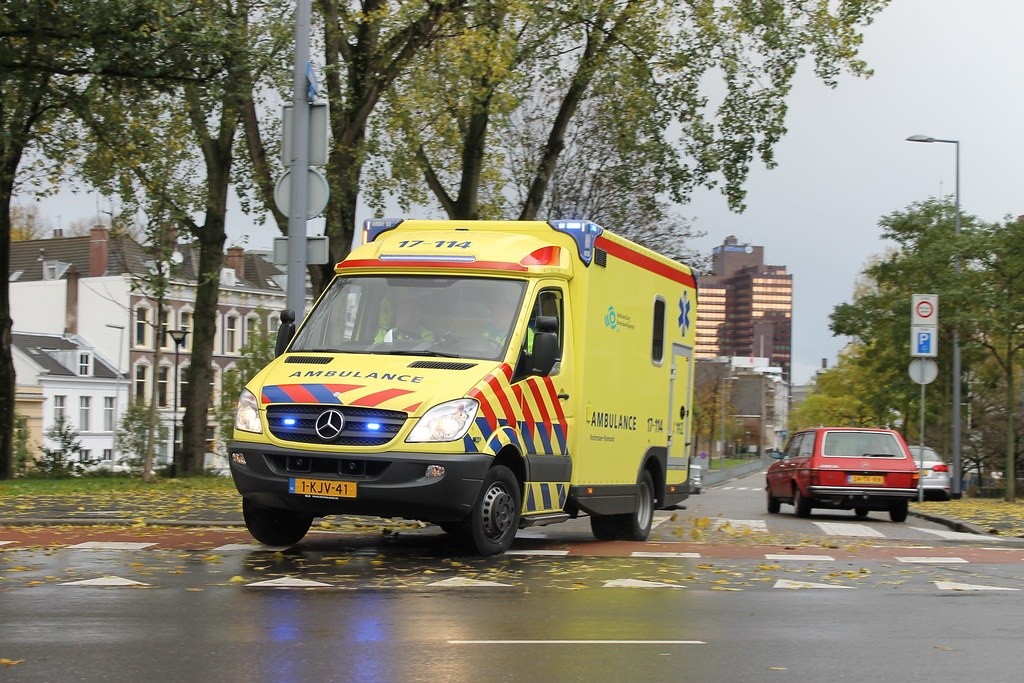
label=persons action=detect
[476,300,537,361]
[381,300,435,355]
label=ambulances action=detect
[226,217,702,557]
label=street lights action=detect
[721,376,741,468]
[164,330,190,481]
[904,133,963,500]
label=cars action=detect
[908,444,954,499]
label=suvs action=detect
[764,425,920,524]
